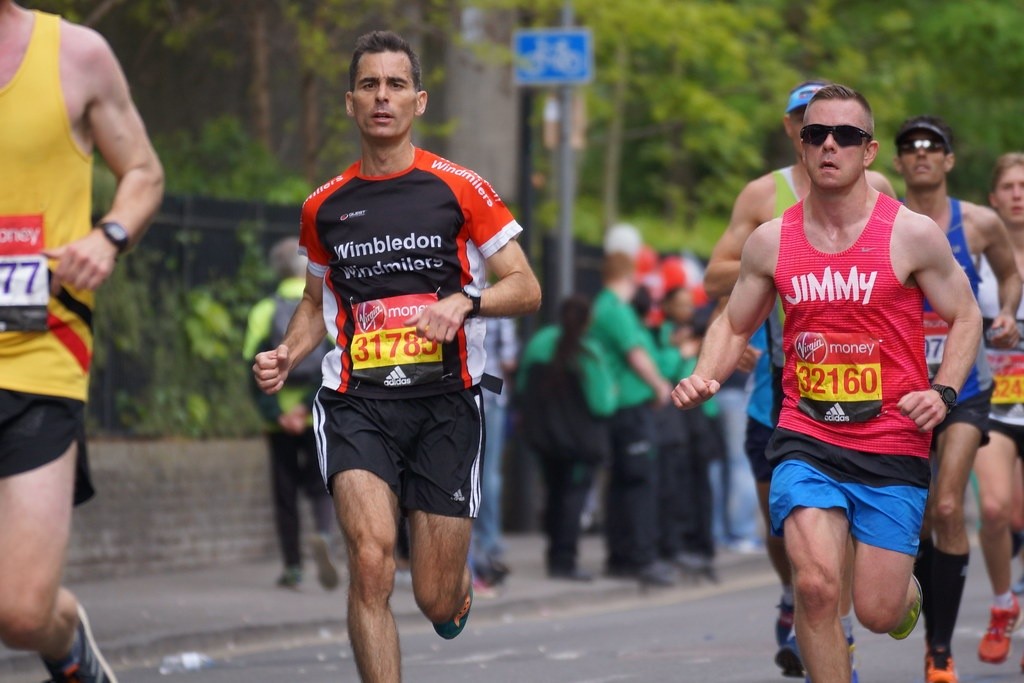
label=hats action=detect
[785,83,826,113]
[893,116,954,155]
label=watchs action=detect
[930,383,958,413]
[93,222,129,263]
[458,284,481,318]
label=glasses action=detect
[896,138,944,154]
[798,122,872,148]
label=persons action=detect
[704,77,1024,683]
[253,31,542,683]
[0,1,166,683]
[671,86,983,683]
[243,220,724,590]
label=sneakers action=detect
[887,589,921,640]
[924,634,958,683]
[774,590,803,677]
[433,586,473,639]
[41,601,119,683]
[978,592,1023,665]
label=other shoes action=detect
[276,563,303,587]
[310,532,341,589]
[847,637,859,682]
